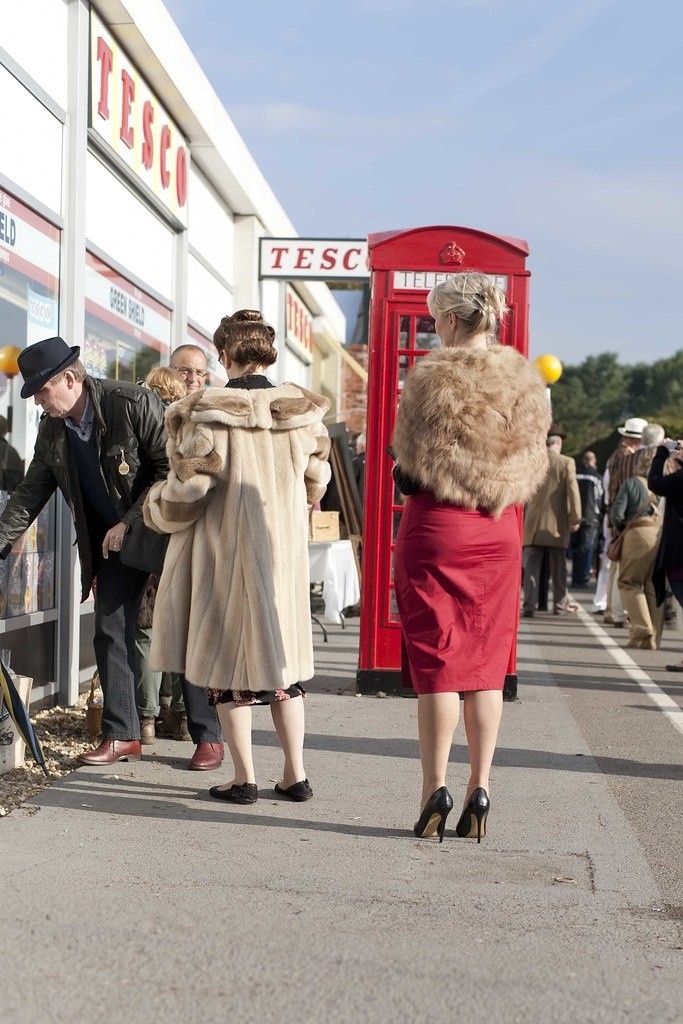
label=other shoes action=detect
[554,610,565,616]
[626,631,653,646]
[521,610,534,617]
[604,617,612,624]
[615,621,623,627]
[209,781,258,805]
[593,609,604,614]
[275,780,313,801]
[665,664,683,672]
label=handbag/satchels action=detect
[118,517,171,574]
[606,535,624,561]
[1,672,33,774]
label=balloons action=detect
[534,354,563,385]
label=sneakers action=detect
[140,716,156,745]
[155,711,191,740]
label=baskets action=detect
[86,668,105,736]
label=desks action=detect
[308,540,360,642]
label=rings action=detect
[114,545,117,547]
[118,546,120,547]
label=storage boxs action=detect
[311,510,340,542]
[0,676,35,776]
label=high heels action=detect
[456,787,490,843]
[413,785,453,844]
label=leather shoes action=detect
[189,741,225,770]
[77,738,143,765]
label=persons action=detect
[0,309,332,805]
[521,417,683,672]
[390,273,552,843]
[348,432,408,614]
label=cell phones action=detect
[670,442,681,451]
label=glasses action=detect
[170,365,207,378]
[217,351,225,366]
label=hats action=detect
[547,423,566,439]
[617,418,649,438]
[17,336,81,400]
